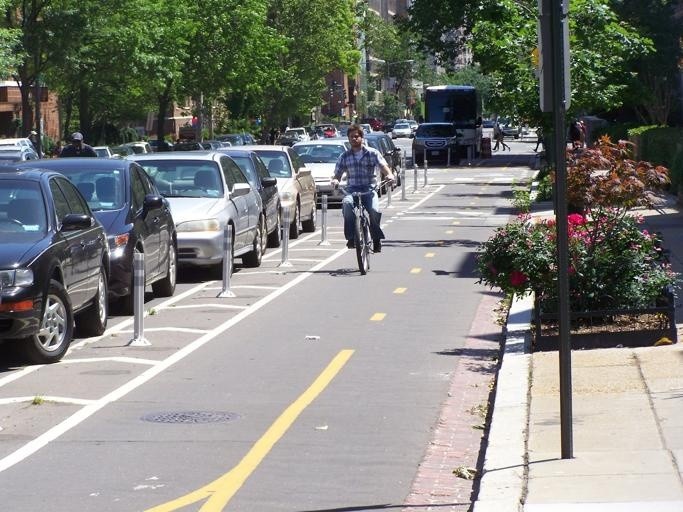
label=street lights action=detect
[387,58,414,94]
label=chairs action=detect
[96,177,119,202]
[194,170,217,190]
[8,199,40,225]
[267,159,283,173]
[77,183,94,202]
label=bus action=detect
[418,83,485,157]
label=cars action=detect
[0,161,112,367]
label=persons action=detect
[26,130,38,153]
[331,124,394,252]
[492,121,545,152]
[571,120,587,148]
[313,127,338,140]
[59,131,97,157]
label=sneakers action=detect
[346,239,355,249]
[371,240,381,253]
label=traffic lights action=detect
[339,88,345,100]
[328,87,333,98]
